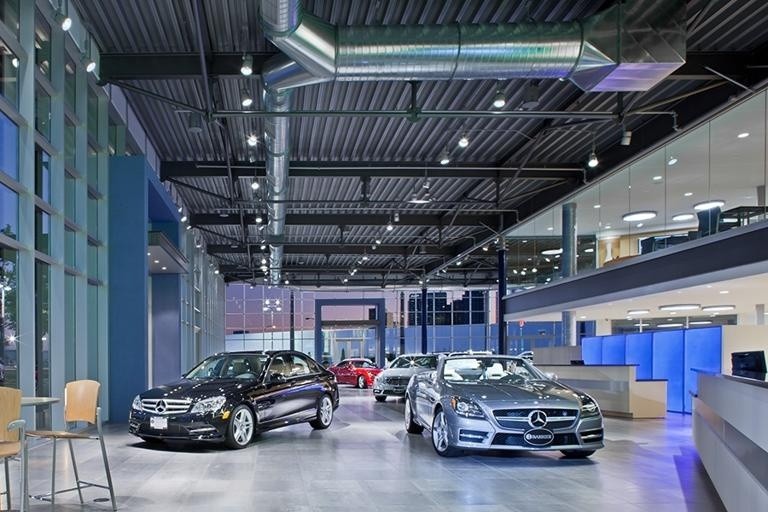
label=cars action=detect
[323,347,610,464]
[126,344,343,452]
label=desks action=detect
[721,204,768,227]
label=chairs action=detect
[640,208,722,254]
[0,379,118,512]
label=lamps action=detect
[622,210,658,222]
[51,8,97,74]
[347,123,480,283]
[587,116,633,168]
[239,50,265,245]
[672,199,726,222]
[493,79,506,108]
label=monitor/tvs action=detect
[731,351,767,380]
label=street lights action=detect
[0,277,13,319]
